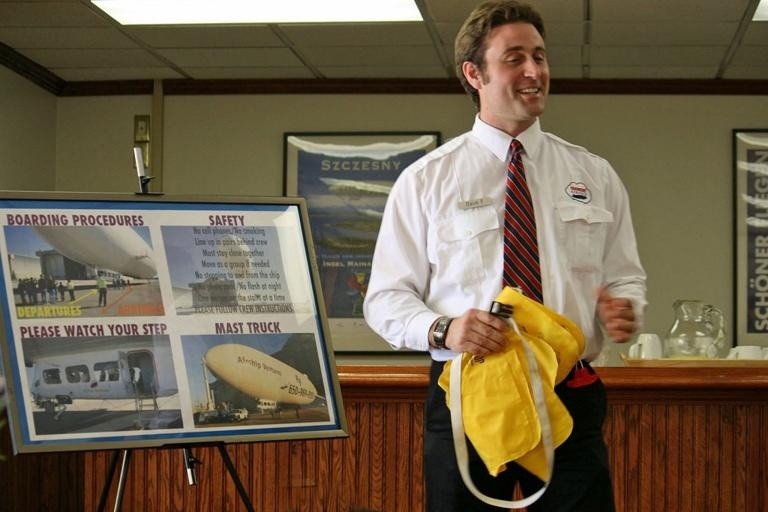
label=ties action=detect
[502,139,543,306]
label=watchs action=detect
[432,317,455,351]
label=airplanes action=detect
[319,175,390,221]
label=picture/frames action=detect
[731,127,768,354]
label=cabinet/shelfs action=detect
[83,366,768,512]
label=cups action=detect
[629,333,662,358]
[727,346,761,360]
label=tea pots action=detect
[662,299,727,358]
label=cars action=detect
[195,408,249,423]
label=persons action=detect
[130,366,144,396]
[97,273,107,307]
[270,404,299,418]
[16,271,75,305]
[113,278,130,289]
[363,0,648,512]
